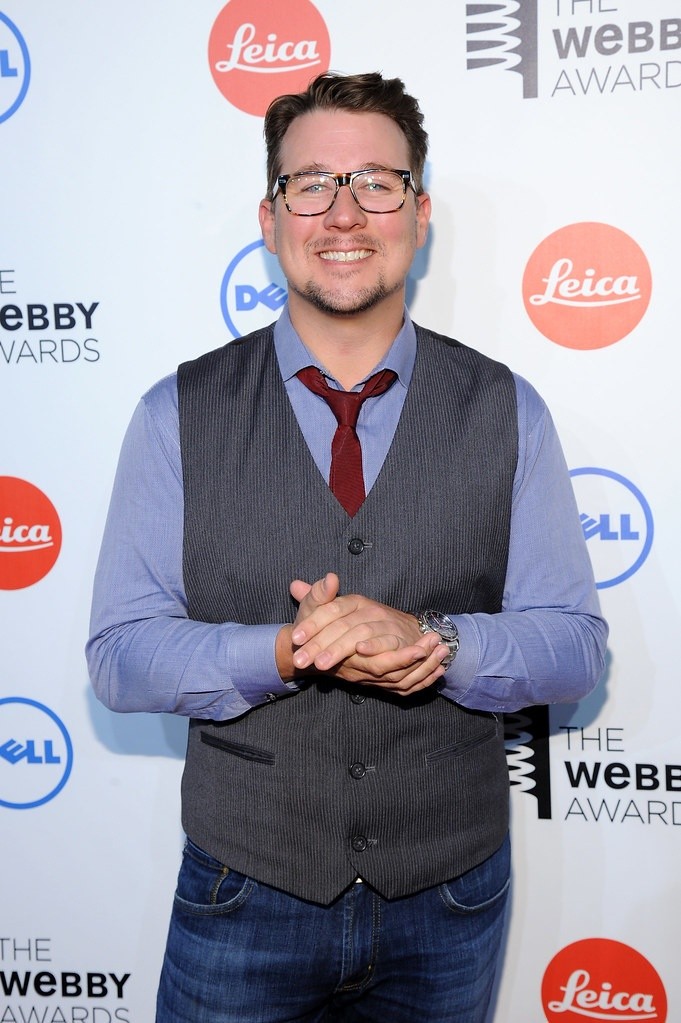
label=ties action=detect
[296,364,398,520]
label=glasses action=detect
[270,168,419,216]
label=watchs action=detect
[403,608,460,671]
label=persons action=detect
[85,74,614,1022]
